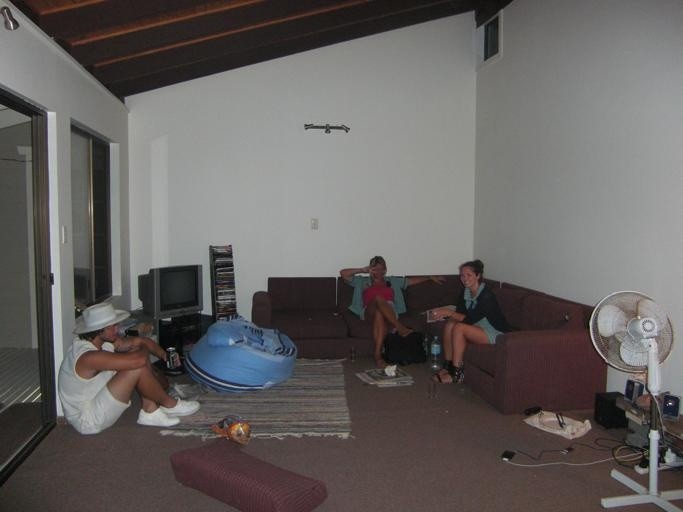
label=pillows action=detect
[168,444,327,512]
[488,285,530,332]
[521,292,585,334]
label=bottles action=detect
[430,336,440,370]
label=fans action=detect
[589,290,681,511]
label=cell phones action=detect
[501,450,515,459]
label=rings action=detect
[433,311,437,315]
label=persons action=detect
[340,255,449,368]
[428,259,512,385]
[56,300,202,435]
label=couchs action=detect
[251,276,606,416]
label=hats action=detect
[73,302,131,334]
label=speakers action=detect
[595,392,627,429]
[663,394,680,422]
[624,380,644,402]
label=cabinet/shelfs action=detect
[129,308,203,352]
[208,245,236,322]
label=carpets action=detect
[155,358,352,439]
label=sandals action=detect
[432,360,452,375]
[433,368,465,384]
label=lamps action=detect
[303,124,351,134]
[0,6,19,31]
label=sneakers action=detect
[159,397,200,416]
[392,326,413,337]
[136,409,180,426]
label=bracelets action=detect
[360,267,362,273]
[426,275,432,282]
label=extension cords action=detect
[634,457,683,475]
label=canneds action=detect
[166,346,178,370]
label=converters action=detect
[664,449,676,463]
[525,407,542,416]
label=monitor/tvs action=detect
[138,265,203,320]
